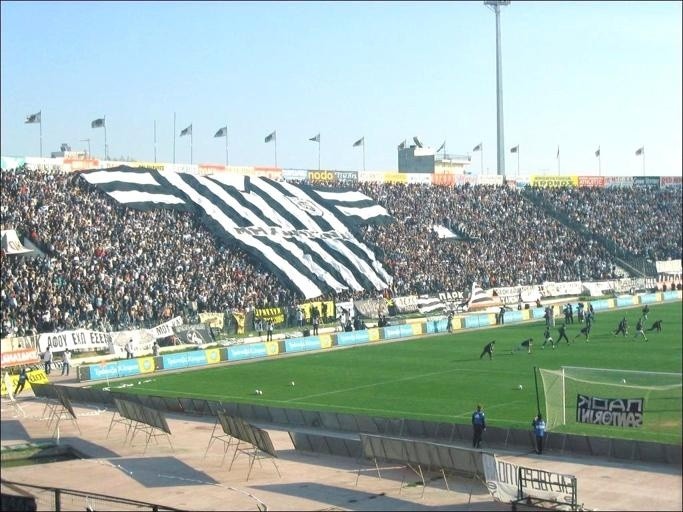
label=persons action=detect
[471,403,486,448]
[0,162,683,396]
[532,413,549,454]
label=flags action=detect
[309,135,320,142]
[472,144,481,151]
[92,118,104,129]
[24,111,40,124]
[635,147,643,155]
[353,137,363,147]
[265,132,276,143]
[214,127,226,137]
[510,146,518,153]
[180,125,191,137]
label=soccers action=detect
[621,378,626,384]
[291,382,295,386]
[255,389,263,395]
[518,384,522,390]
